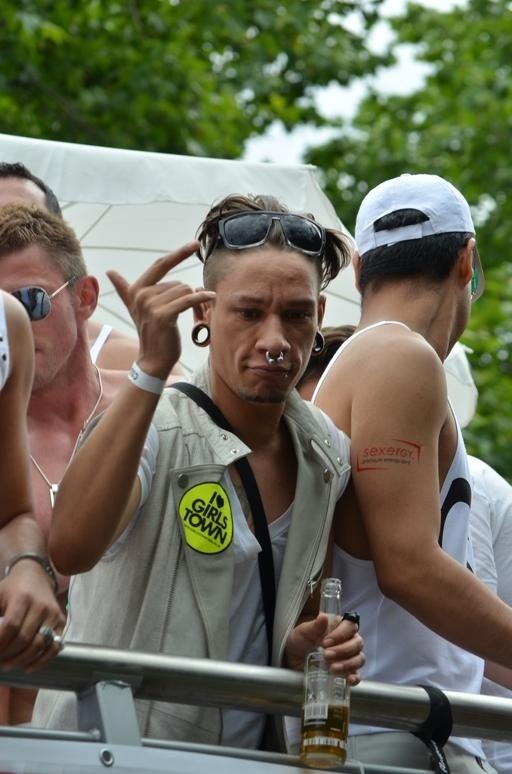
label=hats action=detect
[355,173,485,303]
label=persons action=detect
[291,323,512,774]
[307,174,512,774]
[0,160,185,375]
[33,194,370,755]
[0,200,186,726]
[0,287,67,675]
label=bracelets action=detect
[4,552,58,596]
[126,360,166,395]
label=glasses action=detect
[206,211,326,260]
[11,277,75,322]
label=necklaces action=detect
[29,362,103,513]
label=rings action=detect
[343,610,362,631]
[52,632,64,651]
[38,625,52,647]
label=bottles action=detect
[300,577,358,771]
